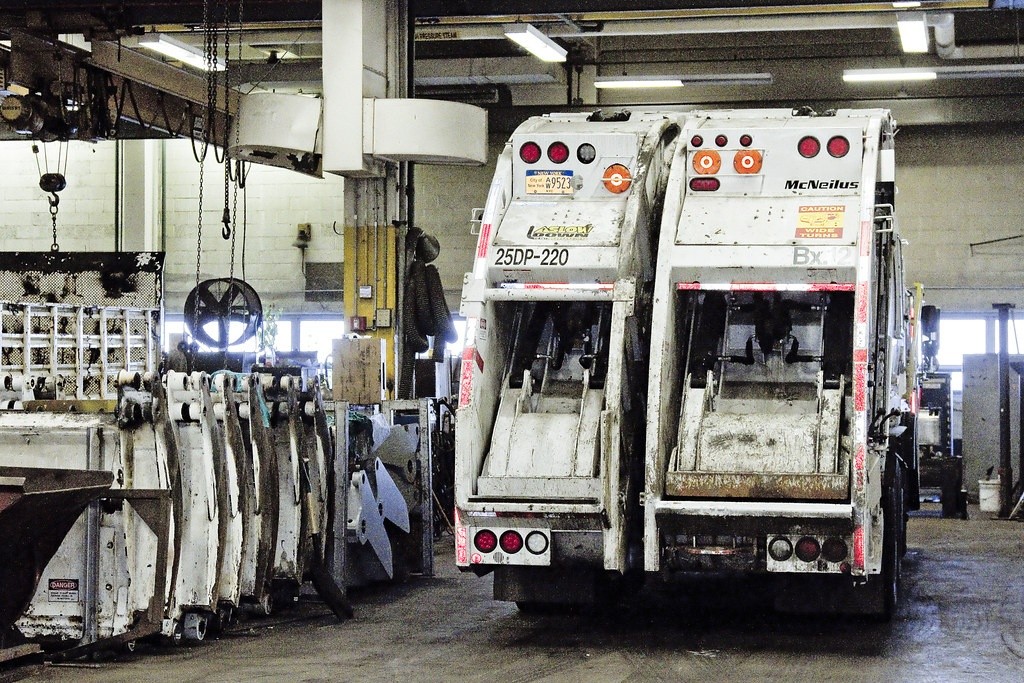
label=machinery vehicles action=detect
[451,108,924,628]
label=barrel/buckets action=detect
[978,479,1001,513]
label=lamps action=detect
[896,11,930,53]
[502,22,568,64]
[136,31,229,73]
